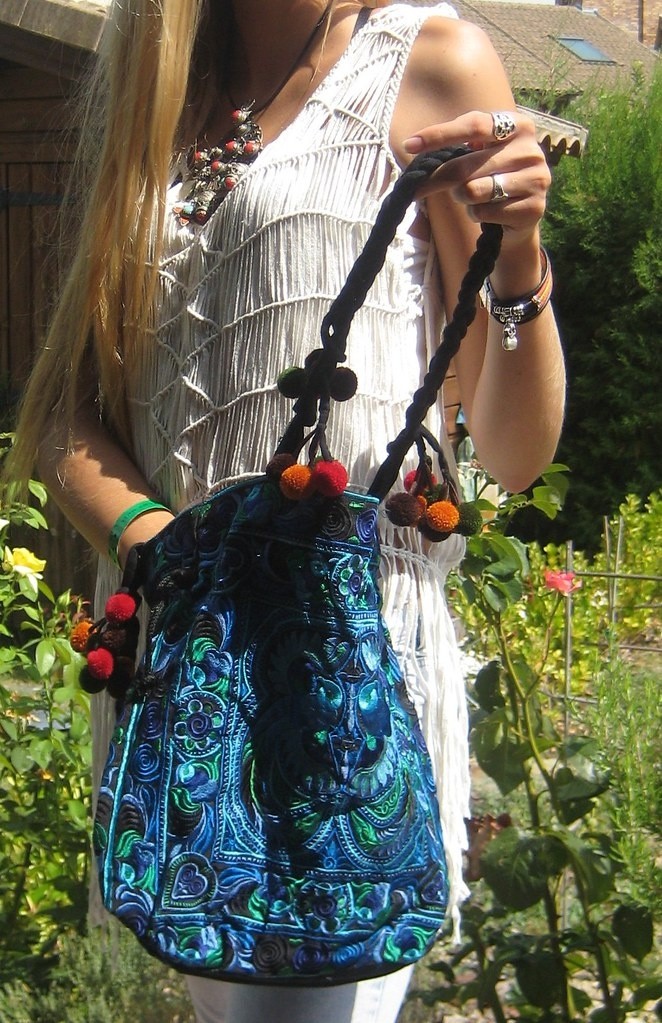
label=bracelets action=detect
[479,244,554,352]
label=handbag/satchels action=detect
[69,139,504,990]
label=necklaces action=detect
[172,0,334,225]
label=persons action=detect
[6,0,567,1023]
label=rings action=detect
[108,499,172,571]
[490,173,509,201]
[489,111,518,140]
